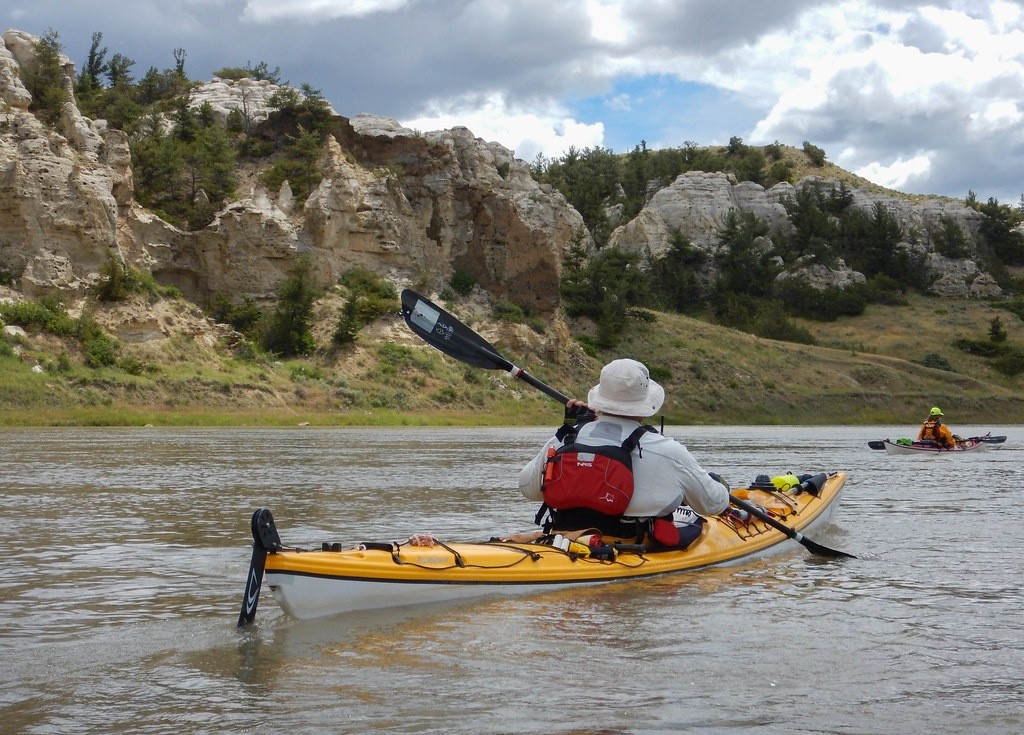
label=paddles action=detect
[400,289,858,560]
[868,435,1007,450]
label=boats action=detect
[881,432,992,456]
[250,474,846,618]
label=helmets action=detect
[930,407,944,416]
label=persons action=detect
[916,407,956,446]
[517,359,730,538]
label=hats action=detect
[587,359,665,417]
